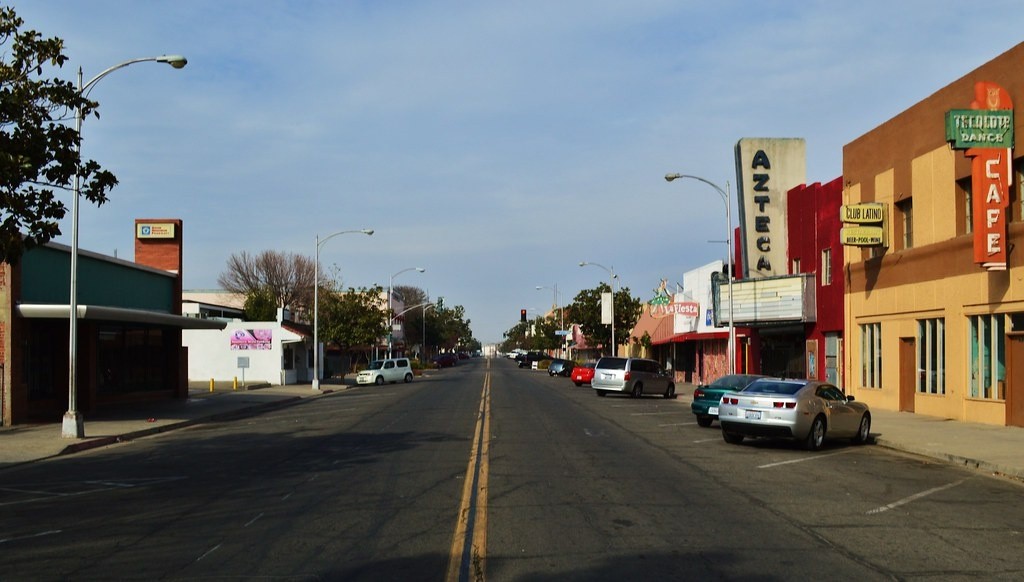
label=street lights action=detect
[664,172,733,374]
[62,54,188,438]
[312,229,374,390]
[389,267,425,359]
[536,286,567,359]
[579,262,615,357]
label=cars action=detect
[503,350,555,368]
[356,358,414,386]
[548,357,675,398]
[430,353,469,369]
[691,375,843,427]
[718,378,871,451]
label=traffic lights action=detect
[521,309,527,322]
